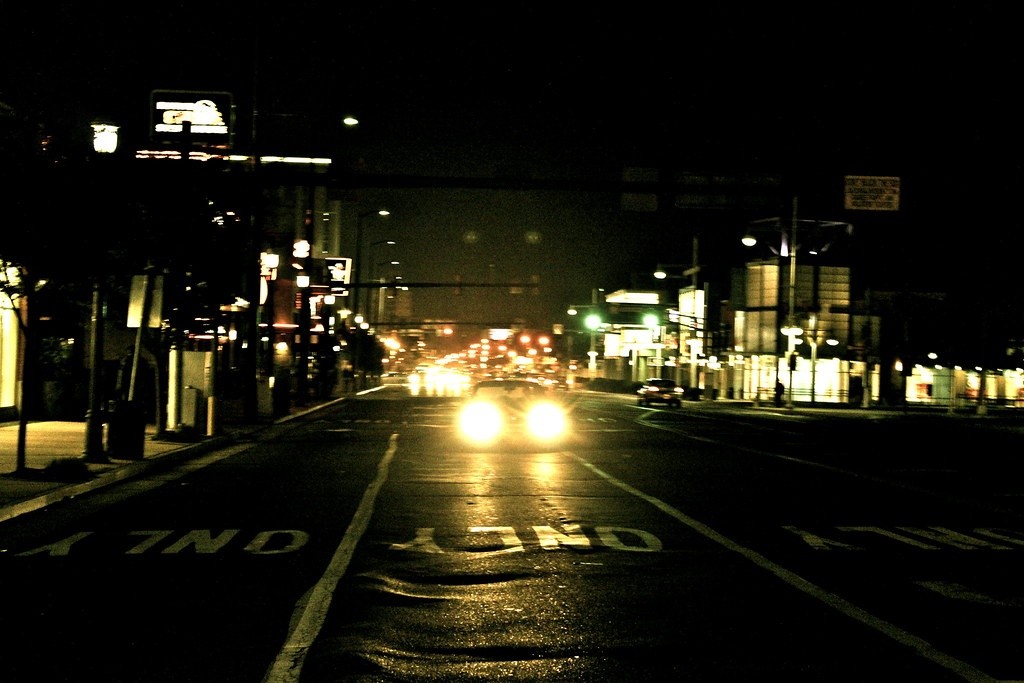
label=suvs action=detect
[637,377,682,409]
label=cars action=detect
[452,377,572,454]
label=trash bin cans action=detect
[105,393,150,462]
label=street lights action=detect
[653,270,698,389]
[739,215,853,407]
[365,239,408,330]
[245,108,362,302]
[566,304,599,378]
[77,105,120,463]
